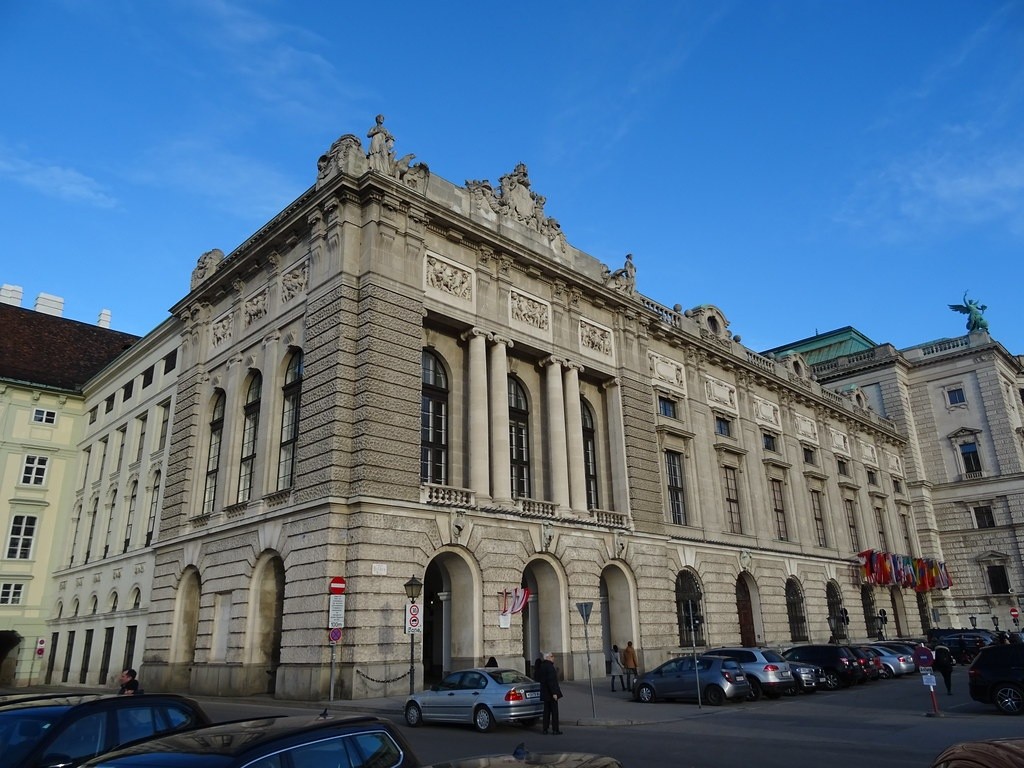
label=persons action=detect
[989,629,1016,647]
[538,652,564,734]
[610,645,626,692]
[626,253,636,295]
[118,669,145,695]
[975,636,985,656]
[964,288,990,335]
[935,643,954,695]
[958,634,971,666]
[623,641,640,691]
[369,114,391,178]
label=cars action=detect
[784,661,826,697]
[857,645,916,679]
[404,667,545,732]
[0,694,625,768]
[632,654,751,707]
[871,641,919,656]
[933,632,1024,664]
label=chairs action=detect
[68,718,98,758]
[469,677,478,688]
[3,722,40,768]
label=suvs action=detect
[968,643,1024,715]
[781,644,885,692]
[702,646,796,701]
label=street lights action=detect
[969,615,977,629]
[874,613,885,640]
[404,574,423,695]
[990,615,1000,631]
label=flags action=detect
[859,549,954,593]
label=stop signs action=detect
[1010,608,1019,618]
[330,576,345,594]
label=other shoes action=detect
[543,730,548,735]
[623,689,626,691]
[612,690,616,692]
[553,731,563,735]
